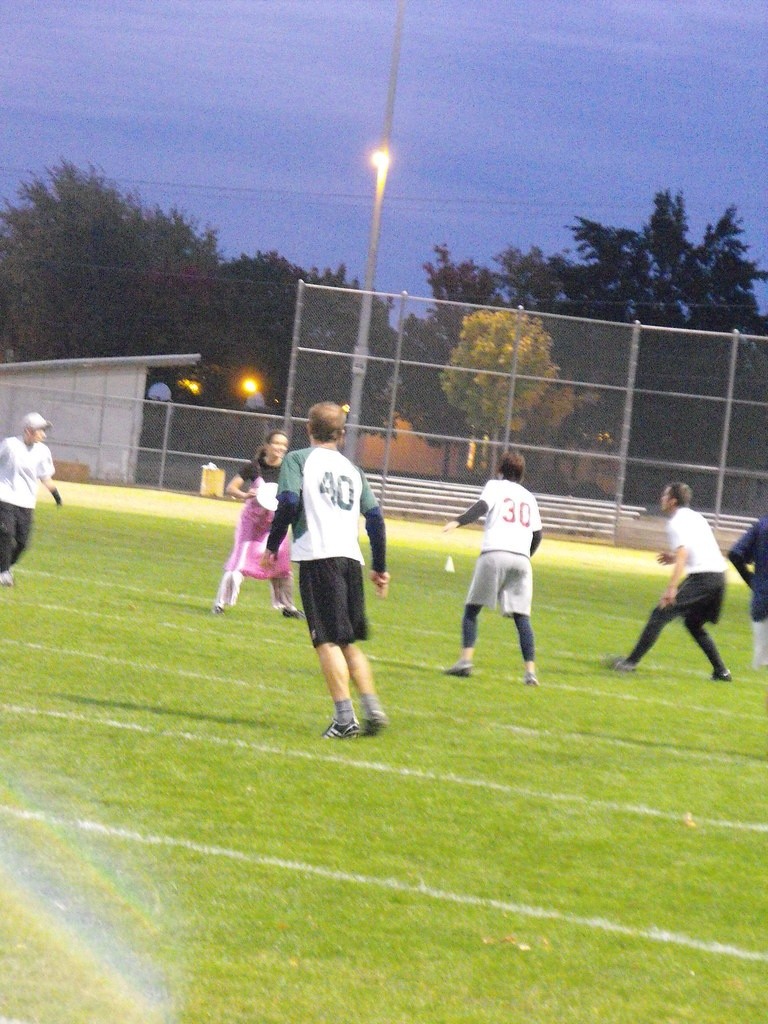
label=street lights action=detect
[341,149,389,463]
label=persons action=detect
[442,451,542,686]
[212,428,307,621]
[605,482,732,682]
[727,513,768,668]
[263,401,392,741]
[0,411,62,586]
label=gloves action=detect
[52,489,64,506]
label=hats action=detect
[21,413,50,441]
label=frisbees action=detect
[257,483,278,511]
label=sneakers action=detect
[321,719,361,741]
[360,710,391,737]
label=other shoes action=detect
[443,664,473,679]
[710,670,733,683]
[0,570,14,587]
[603,656,636,674]
[524,670,540,686]
[283,608,306,620]
[212,606,224,616]
